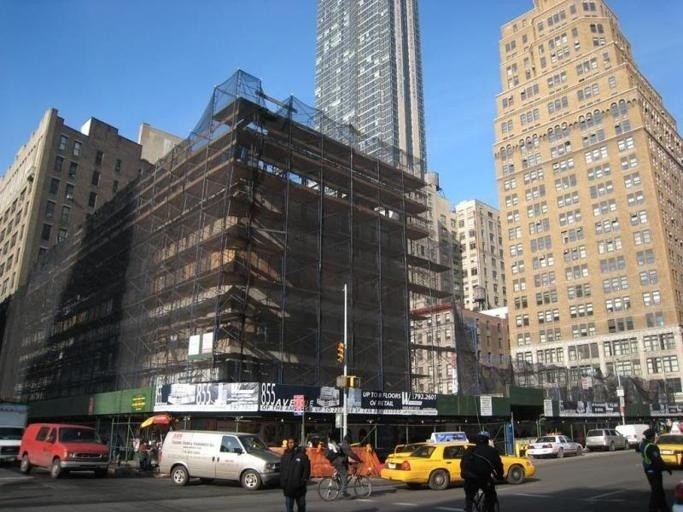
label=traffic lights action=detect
[345,375,360,388]
[336,342,343,363]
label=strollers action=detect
[143,448,159,470]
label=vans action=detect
[586,428,630,451]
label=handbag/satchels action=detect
[463,464,488,482]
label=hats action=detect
[644,429,656,435]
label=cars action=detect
[651,423,682,465]
[378,431,535,490]
[384,437,433,464]
[527,434,583,458]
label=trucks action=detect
[159,428,287,491]
[17,421,110,477]
[0,403,29,468]
[615,424,651,447]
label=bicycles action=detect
[467,471,499,511]
[317,458,372,502]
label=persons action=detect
[329,435,364,497]
[137,439,152,469]
[279,435,311,512]
[638,426,672,512]
[460,430,506,512]
